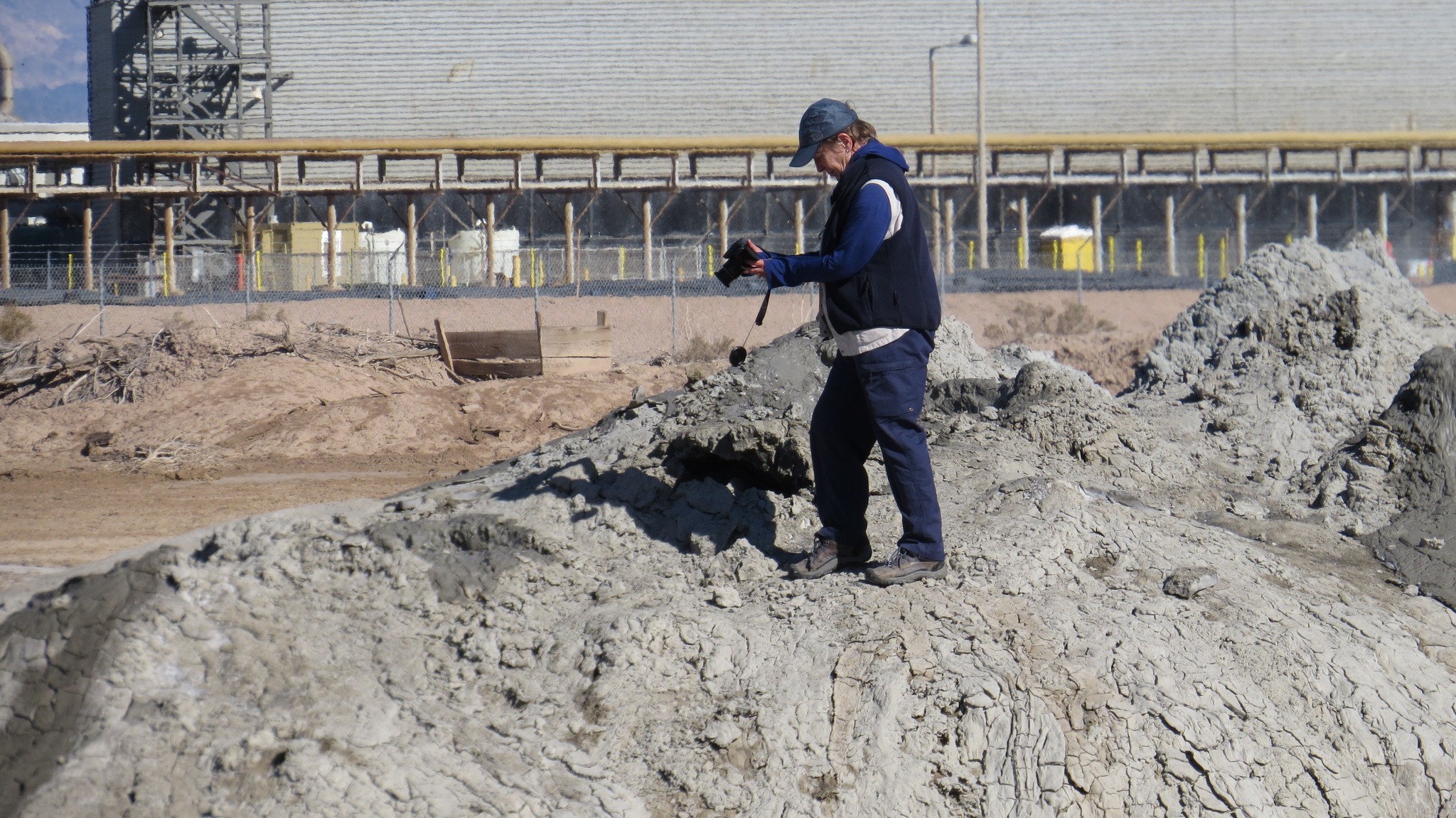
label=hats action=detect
[789,98,858,167]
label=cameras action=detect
[714,237,760,288]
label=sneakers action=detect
[788,532,872,581]
[865,547,947,585]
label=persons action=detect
[738,97,947,585]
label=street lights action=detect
[929,33,978,291]
